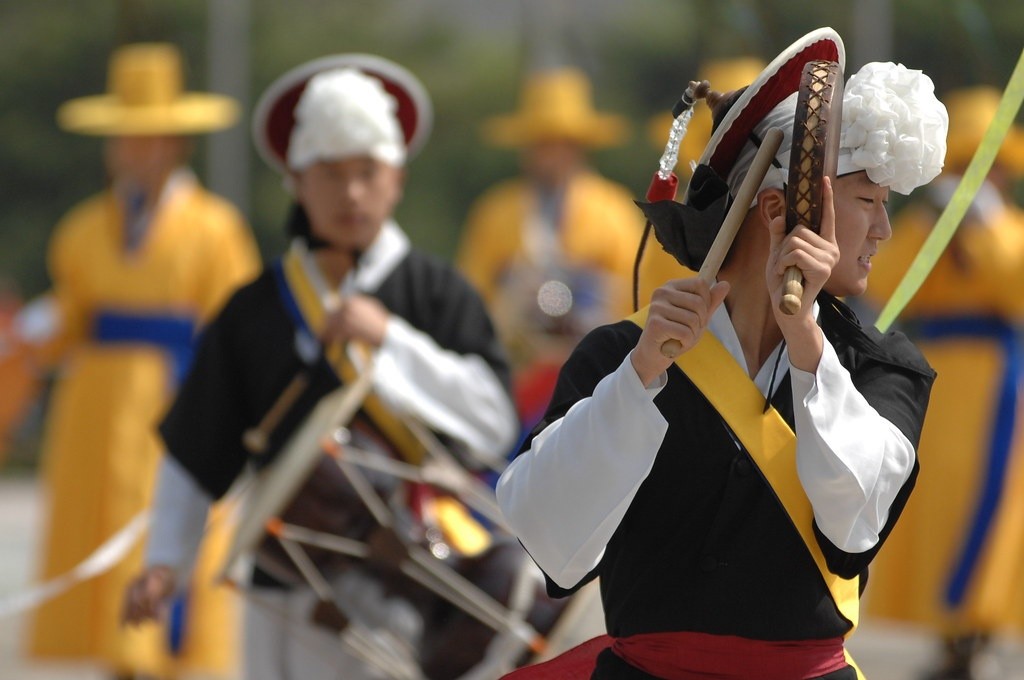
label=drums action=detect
[783,59,846,237]
[206,380,540,679]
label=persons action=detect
[117,52,522,680]
[0,43,1024,680]
[496,25,951,680]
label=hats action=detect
[250,49,435,179]
[54,42,242,138]
[634,26,951,305]
